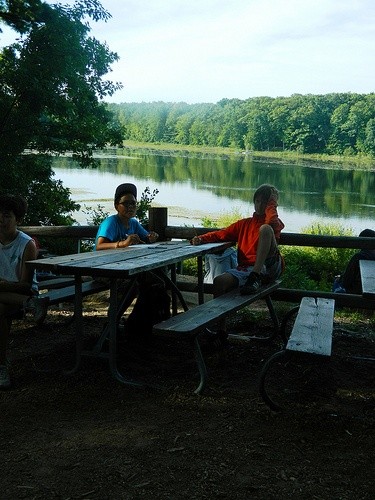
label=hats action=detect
[114,184,137,205]
[335,275,341,278]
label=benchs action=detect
[259,297,335,409]
[152,280,283,393]
[38,275,108,305]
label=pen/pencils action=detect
[124,232,146,244]
[192,224,199,240]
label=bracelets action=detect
[116,240,120,248]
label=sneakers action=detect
[240,272,265,294]
[205,334,228,352]
[0,364,11,388]
[32,297,49,323]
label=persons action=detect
[333,229,375,293]
[91,183,159,341]
[0,195,50,388]
[190,184,285,346]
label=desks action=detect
[24,240,238,373]
[359,259,375,297]
[18,226,98,254]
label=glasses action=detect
[118,200,136,206]
